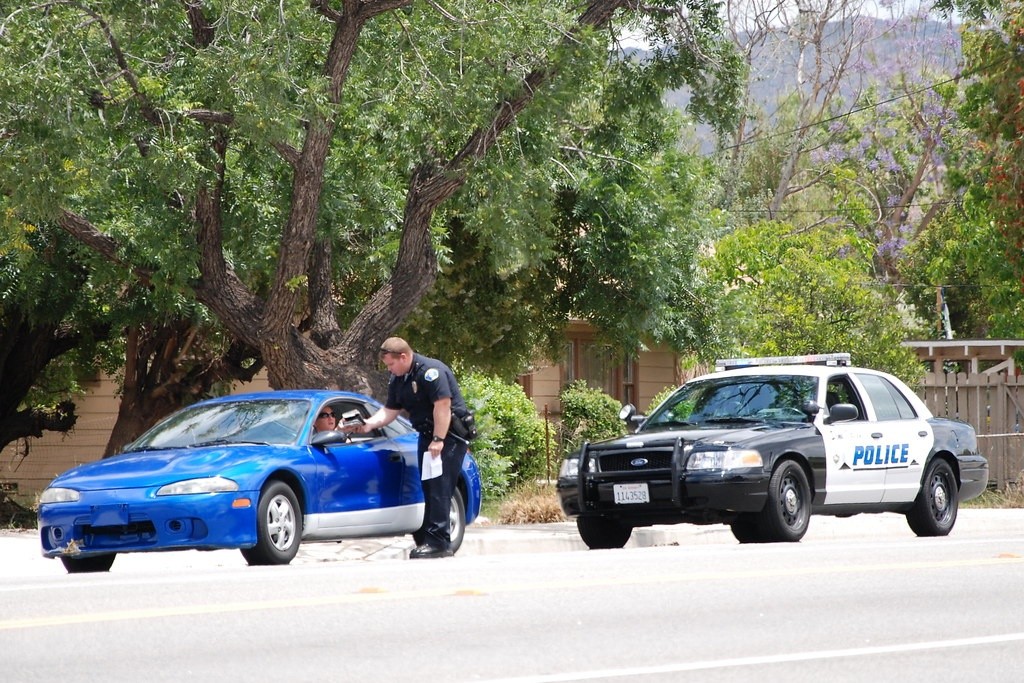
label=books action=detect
[342,409,366,426]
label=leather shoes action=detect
[410,542,454,559]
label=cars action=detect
[556,351,990,551]
[36,388,482,572]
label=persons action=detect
[355,338,477,559]
[313,406,356,442]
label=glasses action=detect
[317,411,336,418]
[379,347,402,355]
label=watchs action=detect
[433,435,444,442]
